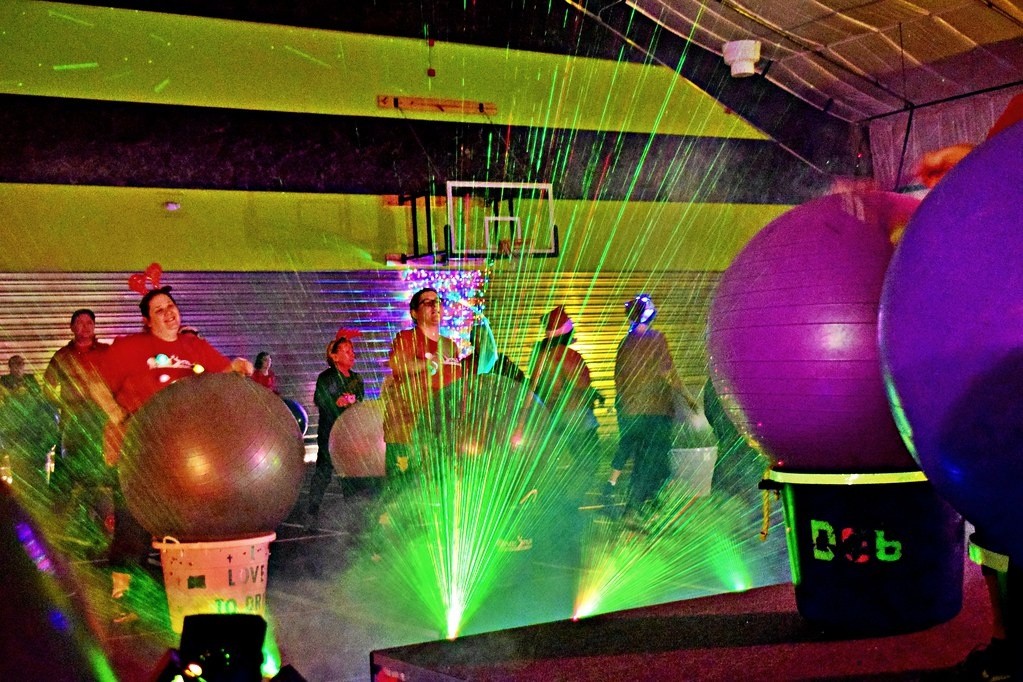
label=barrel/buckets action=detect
[763,457,966,641]
[966,527,1023,651]
[670,445,718,499]
[146,529,276,642]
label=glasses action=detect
[419,298,441,307]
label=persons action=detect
[0,355,41,397]
[304,340,365,530]
[386,289,461,488]
[463,326,525,383]
[87,287,254,625]
[536,307,606,473]
[44,309,111,503]
[252,352,283,397]
[616,297,698,529]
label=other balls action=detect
[875,121,1022,549]
[116,371,307,545]
[327,398,388,479]
[414,371,556,499]
[700,188,925,473]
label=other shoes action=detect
[352,528,369,541]
[603,482,616,497]
[305,511,317,536]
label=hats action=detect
[541,305,572,338]
[626,295,653,322]
